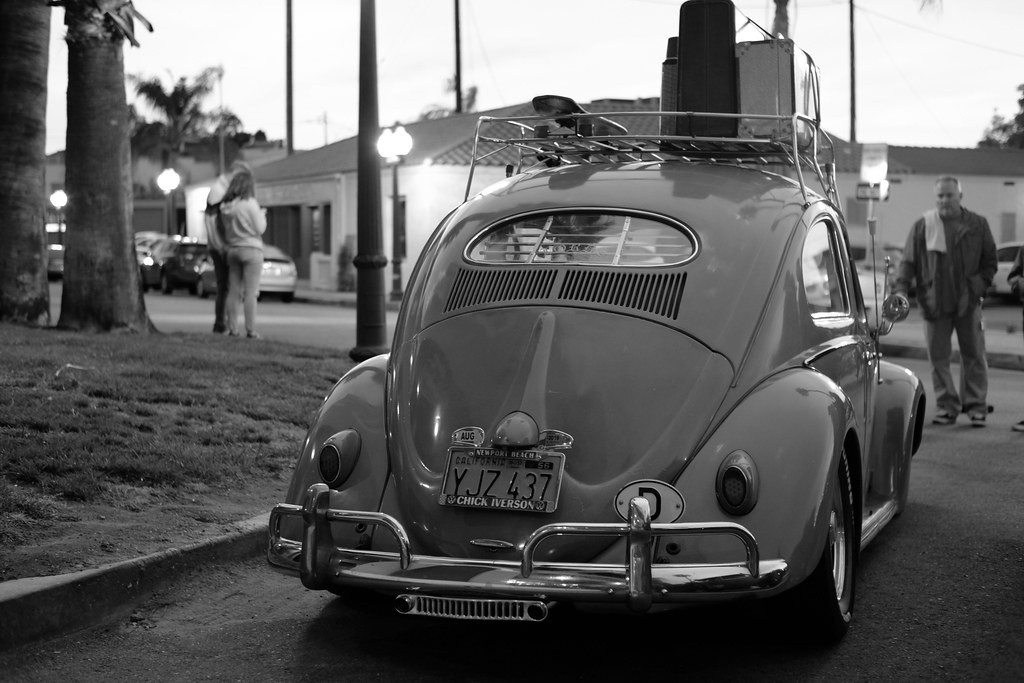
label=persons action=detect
[1006,243,1024,431]
[204,161,267,339]
[890,176,999,428]
[338,235,358,292]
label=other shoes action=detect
[1012,421,1024,430]
[229,330,240,337]
[247,333,260,339]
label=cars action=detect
[47,224,68,277]
[132,230,296,304]
[983,242,1024,295]
[268,110,926,662]
[856,265,893,335]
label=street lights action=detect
[50,189,67,244]
[156,168,180,234]
[377,125,412,303]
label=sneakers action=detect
[971,414,986,425]
[932,410,957,424]
